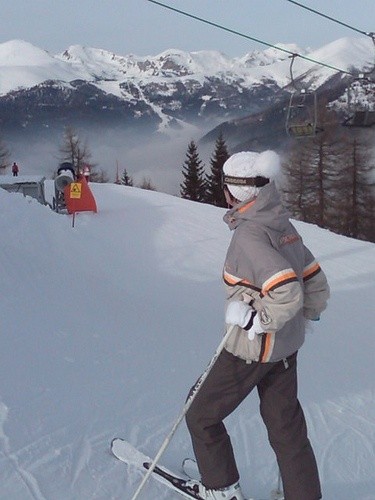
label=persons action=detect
[181,151,331,500]
[11,162,19,176]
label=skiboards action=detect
[110,435,203,500]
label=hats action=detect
[223,150,280,204]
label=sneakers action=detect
[200,484,242,499]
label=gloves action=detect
[225,303,260,340]
